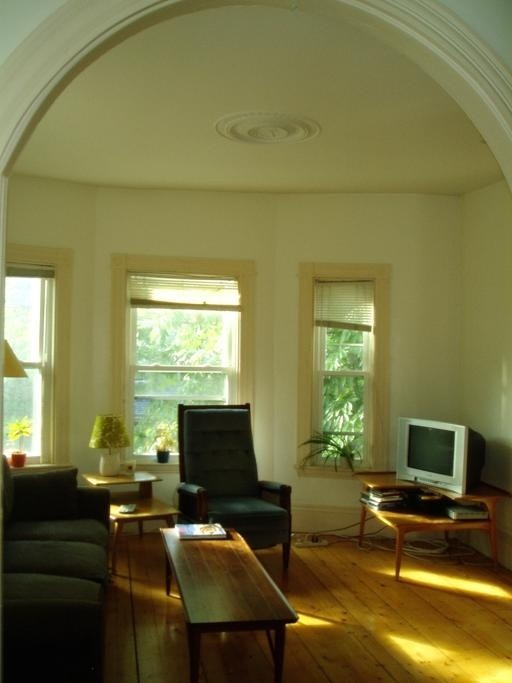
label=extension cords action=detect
[295,539,329,547]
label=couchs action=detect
[1,452,113,682]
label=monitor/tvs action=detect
[395,417,486,495]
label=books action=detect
[359,488,403,510]
[174,523,226,539]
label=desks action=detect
[81,470,183,576]
[352,479,512,580]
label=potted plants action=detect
[6,414,36,467]
[297,424,364,474]
[149,427,178,462]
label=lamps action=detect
[2,339,30,379]
[89,412,132,476]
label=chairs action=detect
[174,399,294,570]
